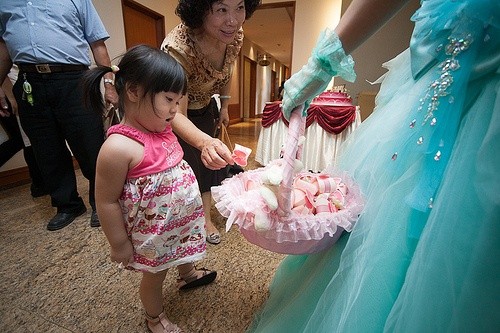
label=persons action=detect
[84,45,218,333]
[245,0,500,333]
[0,0,121,231]
[158,0,259,244]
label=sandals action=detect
[143,310,185,333]
[175,267,218,290]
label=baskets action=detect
[210,104,363,254]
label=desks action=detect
[255,101,361,173]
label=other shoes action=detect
[207,231,221,244]
[30,181,50,198]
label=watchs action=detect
[103,78,114,85]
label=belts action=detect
[19,60,89,75]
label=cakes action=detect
[313,90,353,105]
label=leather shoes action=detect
[47,197,86,230]
[90,209,101,227]
